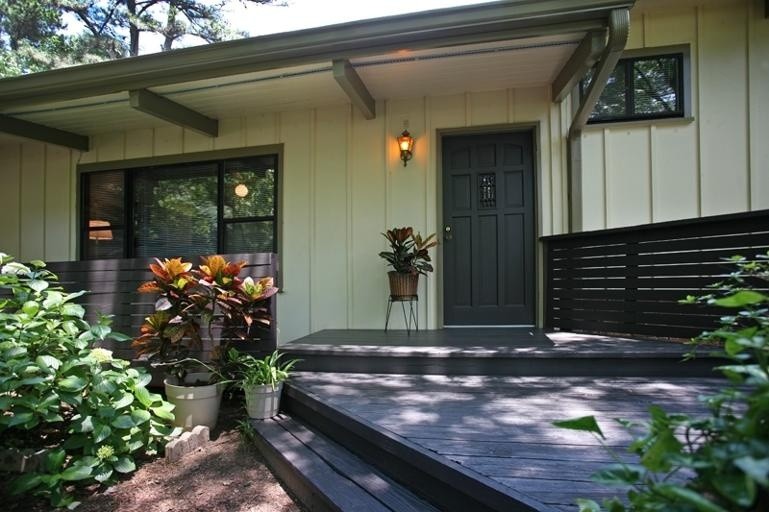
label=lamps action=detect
[396,128,416,166]
[90,220,113,260]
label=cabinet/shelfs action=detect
[383,295,418,337]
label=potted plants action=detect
[379,226,440,300]
[209,348,305,420]
[129,254,280,432]
